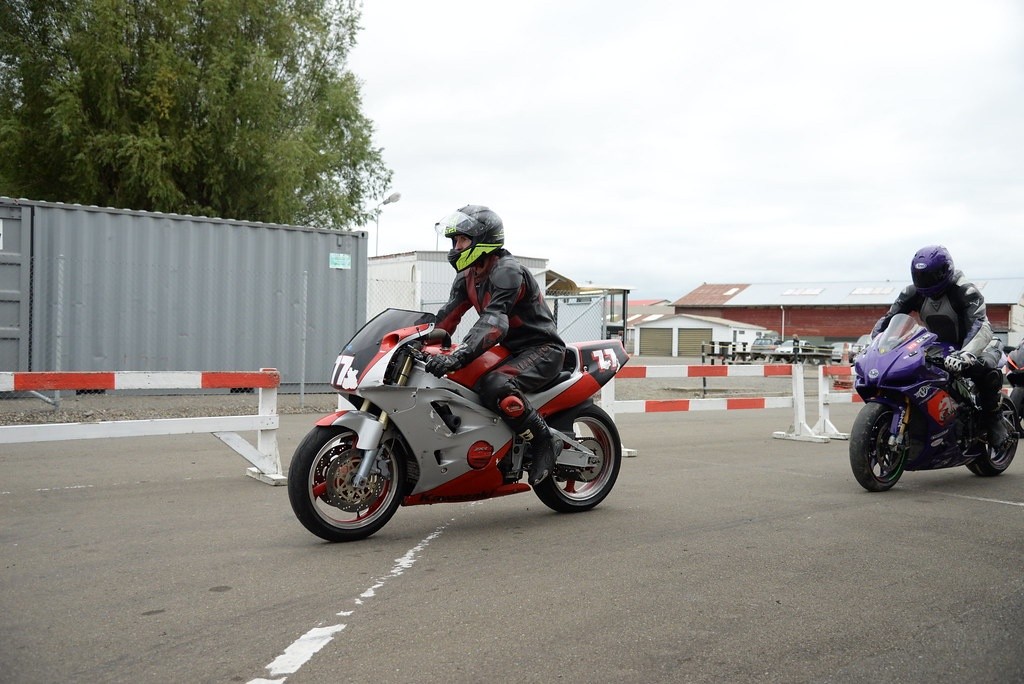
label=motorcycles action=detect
[285,308,632,542]
[1001,341,1024,440]
[847,314,1019,493]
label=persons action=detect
[789,334,800,364]
[871,245,1006,448]
[419,205,567,487]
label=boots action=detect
[985,396,1008,448]
[516,408,563,485]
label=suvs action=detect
[849,333,871,365]
[750,337,783,360]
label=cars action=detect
[830,342,854,363]
[774,339,814,363]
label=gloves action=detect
[424,354,461,378]
[944,350,976,374]
[853,344,870,364]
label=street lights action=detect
[374,191,400,257]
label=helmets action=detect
[911,245,955,298]
[446,204,505,273]
